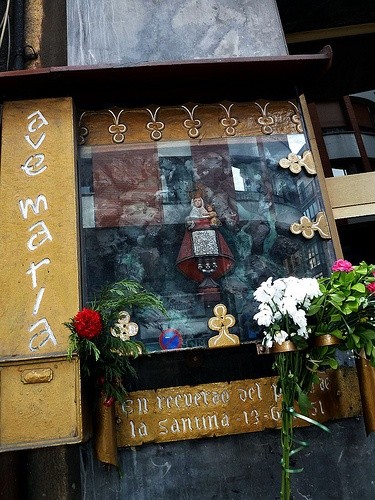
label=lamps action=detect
[175,190,238,304]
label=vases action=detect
[354,356,375,439]
[96,396,118,466]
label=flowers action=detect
[250,257,326,500]
[60,279,169,404]
[300,259,375,401]
